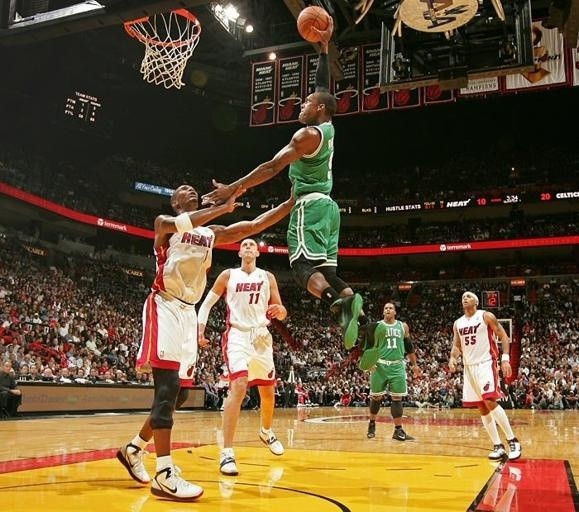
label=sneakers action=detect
[332,293,363,351]
[508,440,522,459]
[259,430,284,455]
[151,465,203,501]
[367,422,375,437]
[358,322,386,370]
[116,441,150,483]
[488,444,506,459]
[392,428,414,440]
[219,448,239,474]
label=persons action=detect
[197,238,287,474]
[343,303,423,441]
[1,248,579,410]
[200,15,387,369]
[448,291,523,459]
[115,185,296,499]
[0,146,578,249]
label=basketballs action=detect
[298,7,329,43]
